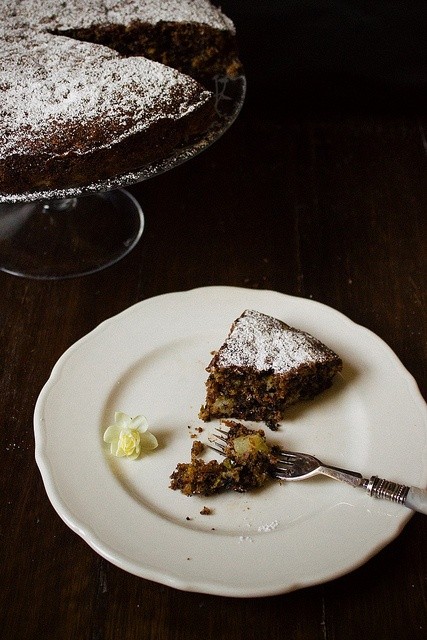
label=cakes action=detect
[0,0,236,193]
[199,308,342,432]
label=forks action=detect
[206,420,427,524]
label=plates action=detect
[31,284,426,599]
